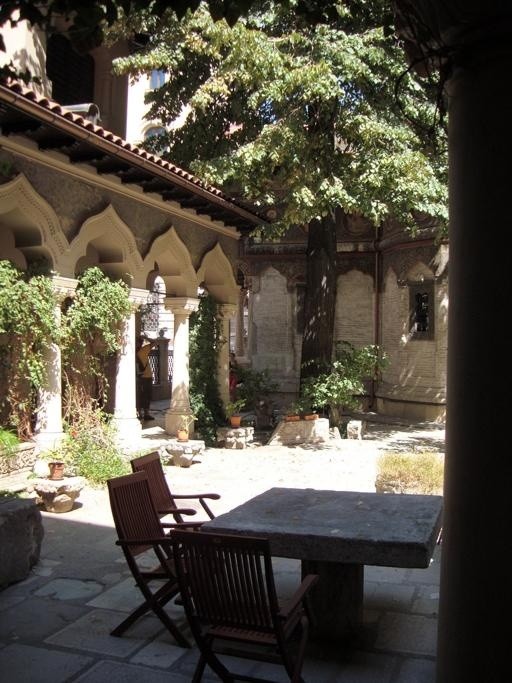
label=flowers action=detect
[176,414,198,442]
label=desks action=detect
[202,485,443,648]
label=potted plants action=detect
[283,396,320,422]
[38,448,66,480]
[224,399,246,428]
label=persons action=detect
[230,367,237,393]
[136,333,172,419]
[229,351,241,375]
